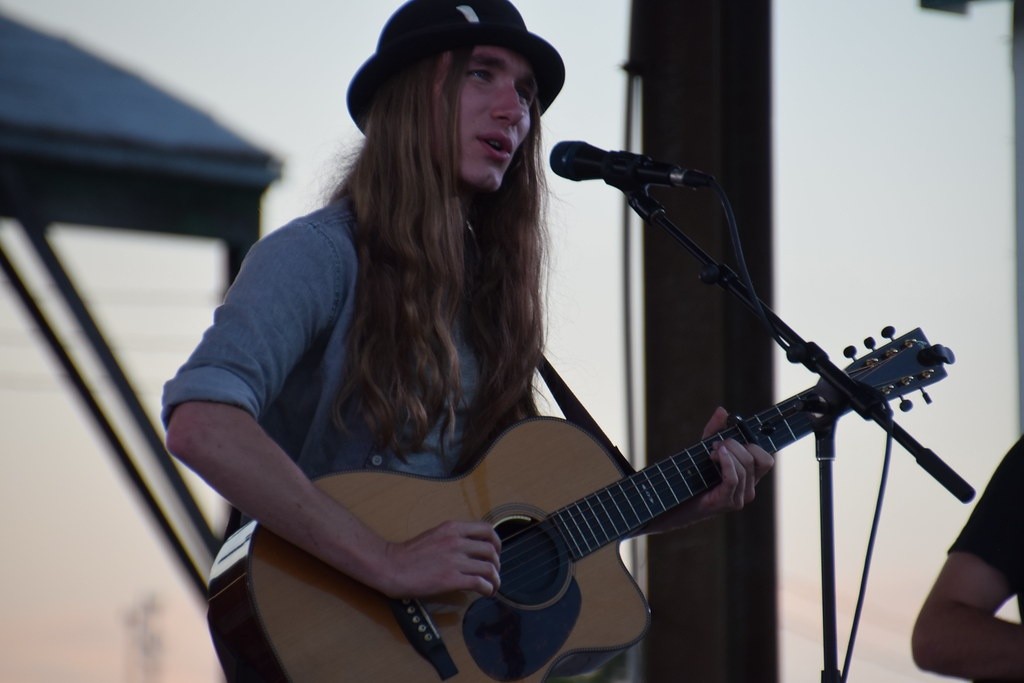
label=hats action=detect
[348,0,566,138]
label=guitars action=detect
[207,328,954,683]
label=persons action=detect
[157,1,774,652]
[910,369,1024,680]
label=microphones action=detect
[550,140,714,194]
